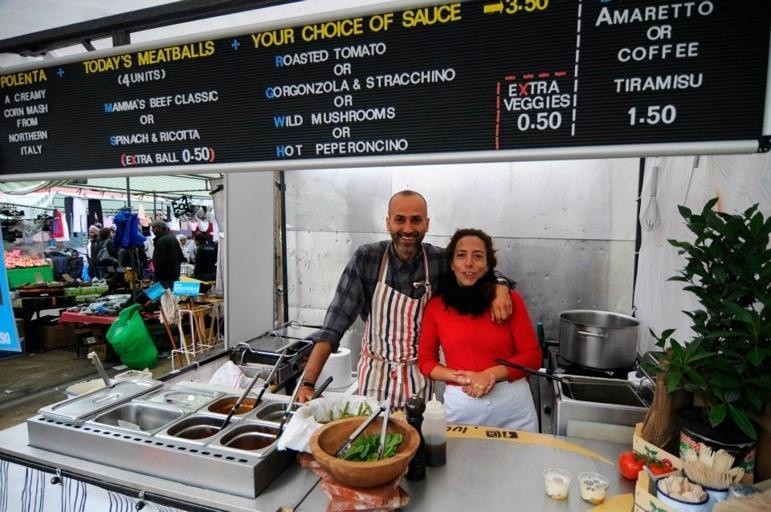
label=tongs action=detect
[333,396,392,462]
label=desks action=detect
[58,296,221,363]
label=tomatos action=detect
[620,451,678,481]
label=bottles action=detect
[422,392,447,467]
[405,392,427,481]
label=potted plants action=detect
[645,194,771,490]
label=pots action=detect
[556,308,642,372]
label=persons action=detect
[67,249,84,277]
[88,220,218,291]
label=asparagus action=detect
[329,401,374,421]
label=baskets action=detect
[64,286,108,297]
[172,281,200,297]
[143,282,166,303]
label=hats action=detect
[149,220,166,228]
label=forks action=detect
[683,439,745,486]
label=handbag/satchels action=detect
[97,248,118,266]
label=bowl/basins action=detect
[542,466,574,501]
[310,415,421,488]
[653,475,710,512]
[577,471,610,506]
[680,468,730,504]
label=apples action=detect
[3,250,48,269]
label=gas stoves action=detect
[546,345,640,382]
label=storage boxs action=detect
[33,313,76,351]
